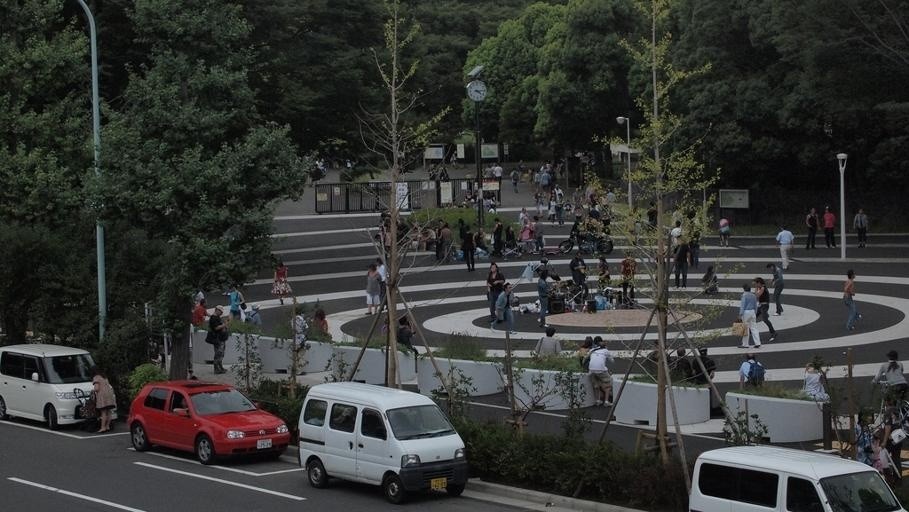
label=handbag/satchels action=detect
[733,323,747,336]
[889,429,906,445]
[205,332,220,344]
[241,300,247,309]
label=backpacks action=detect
[744,361,764,385]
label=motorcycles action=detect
[559,214,613,254]
[586,211,611,234]
[429,167,451,180]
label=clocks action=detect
[467,80,487,101]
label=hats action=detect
[216,305,224,312]
[252,303,260,310]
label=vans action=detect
[297,381,469,504]
[0,343,118,430]
[689,444,908,511]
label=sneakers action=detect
[596,400,612,407]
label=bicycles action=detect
[871,380,909,437]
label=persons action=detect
[766,261,785,316]
[805,207,822,250]
[801,357,831,403]
[288,306,309,375]
[851,208,870,249]
[751,277,777,343]
[739,354,766,391]
[88,365,117,434]
[855,408,903,492]
[822,206,837,250]
[869,350,908,423]
[735,283,762,348]
[270,261,293,306]
[152,283,262,380]
[841,269,864,333]
[775,226,794,272]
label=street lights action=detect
[616,117,633,216]
[836,154,847,260]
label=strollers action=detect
[702,271,720,296]
[73,387,114,434]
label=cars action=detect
[126,379,291,465]
